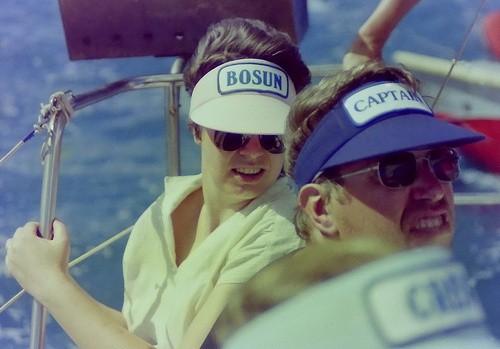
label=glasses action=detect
[326,148,461,190]
[214,130,286,154]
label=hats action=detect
[222,247,500,349]
[188,59,297,135]
[294,80,486,187]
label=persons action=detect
[7,15,311,349]
[340,0,420,74]
[209,241,500,349]
[284,59,486,248]
